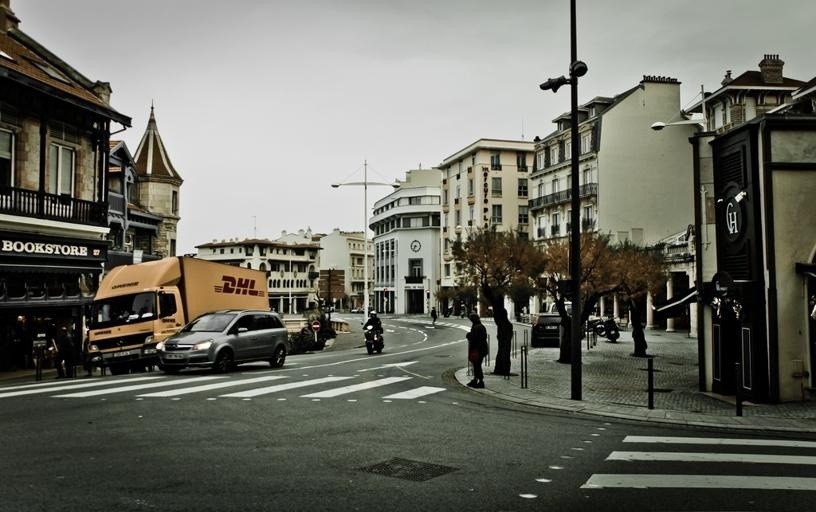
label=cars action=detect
[320,304,364,315]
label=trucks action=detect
[82,252,272,376]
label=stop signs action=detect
[311,320,321,333]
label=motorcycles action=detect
[600,313,620,343]
[359,321,384,355]
[584,315,606,337]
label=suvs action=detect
[529,311,570,348]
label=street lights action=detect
[540,57,588,400]
[648,81,709,133]
[329,159,402,328]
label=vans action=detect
[548,300,586,339]
[152,307,291,375]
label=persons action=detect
[464,310,490,387]
[431,306,439,325]
[52,342,65,379]
[62,337,73,378]
[82,328,95,377]
[365,310,384,338]
